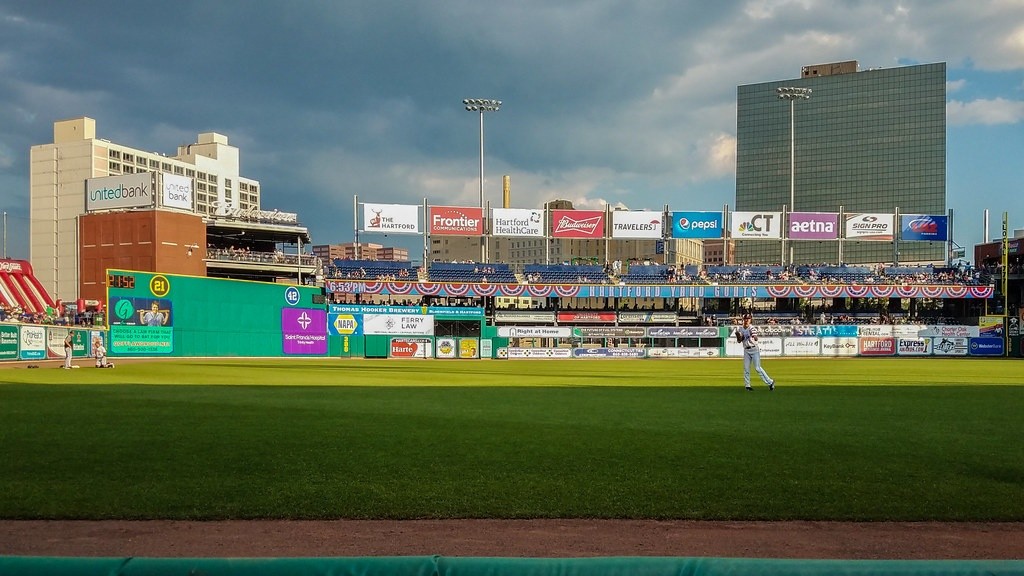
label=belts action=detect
[745,346,755,349]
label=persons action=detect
[731,314,961,325]
[576,276,607,284]
[0,301,105,327]
[139,301,169,326]
[605,259,622,279]
[334,299,910,312]
[735,314,776,392]
[95,341,115,368]
[527,272,542,283]
[970,300,1020,317]
[432,258,473,263]
[566,258,600,267]
[206,243,315,266]
[474,265,497,283]
[628,257,1023,287]
[336,267,410,281]
[63,330,75,369]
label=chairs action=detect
[328,266,986,285]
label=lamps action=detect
[187,242,199,256]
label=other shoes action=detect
[769,381,776,392]
[746,387,754,392]
[107,362,115,368]
[63,366,71,369]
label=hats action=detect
[743,314,752,319]
[70,331,74,333]
